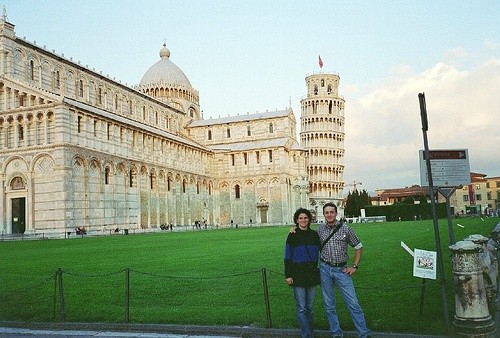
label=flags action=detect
[319,57,323,68]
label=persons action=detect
[198,221,201,227]
[195,220,198,228]
[170,221,173,231]
[160,222,169,230]
[283,208,323,338]
[484,208,487,216]
[76,226,87,235]
[205,220,207,229]
[456,210,459,216]
[290,203,375,338]
[461,210,464,214]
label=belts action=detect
[320,259,347,267]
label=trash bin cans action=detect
[448,239,498,338]
[125,229,128,235]
[465,234,497,302]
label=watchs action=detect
[353,265,358,268]
